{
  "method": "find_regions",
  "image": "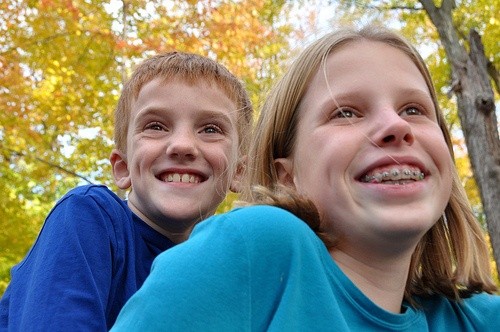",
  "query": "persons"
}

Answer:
[0,51,254,332]
[109,26,500,332]
